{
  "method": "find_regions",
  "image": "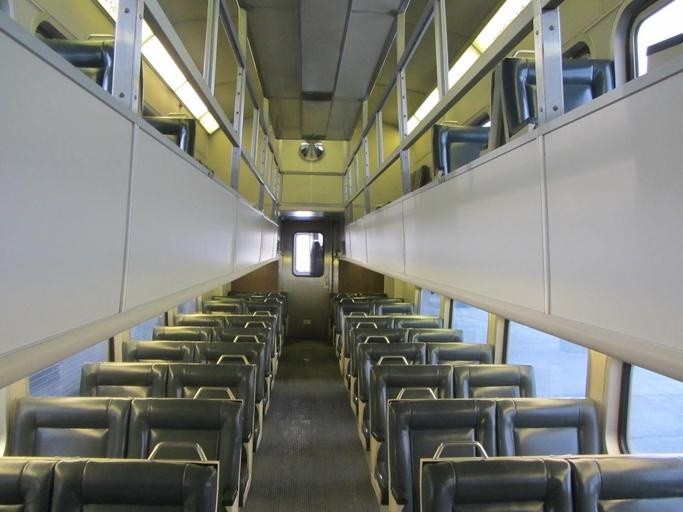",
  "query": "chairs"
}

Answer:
[40,40,114,94]
[437,127,489,175]
[514,56,614,126]
[144,115,194,156]
